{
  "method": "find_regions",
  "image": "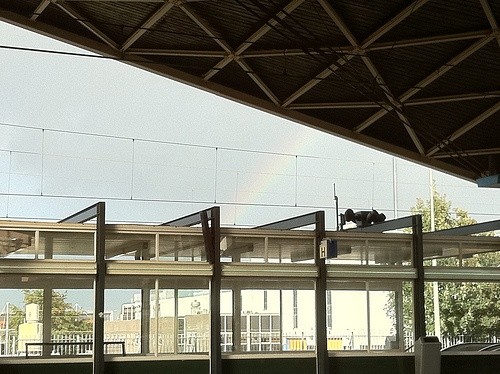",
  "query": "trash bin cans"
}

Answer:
[414,335,442,373]
